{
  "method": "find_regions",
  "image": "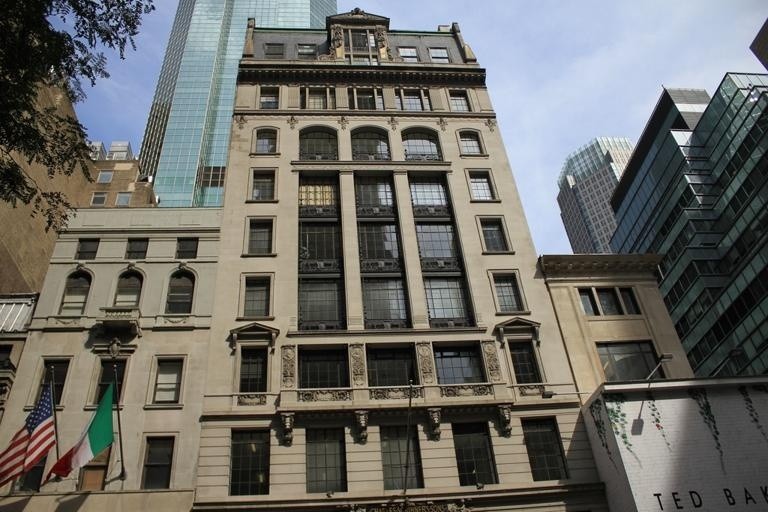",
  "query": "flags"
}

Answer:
[42,380,123,488]
[1,378,60,487]
